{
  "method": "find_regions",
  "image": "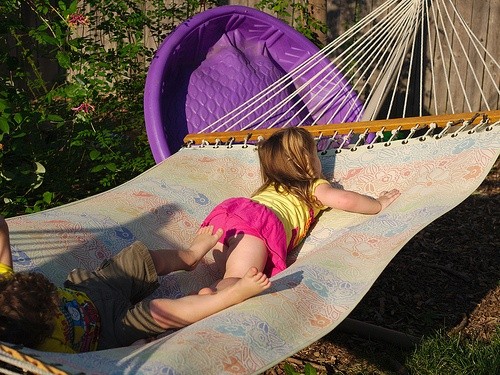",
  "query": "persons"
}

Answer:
[198,127,401,294]
[0,215,270,354]
